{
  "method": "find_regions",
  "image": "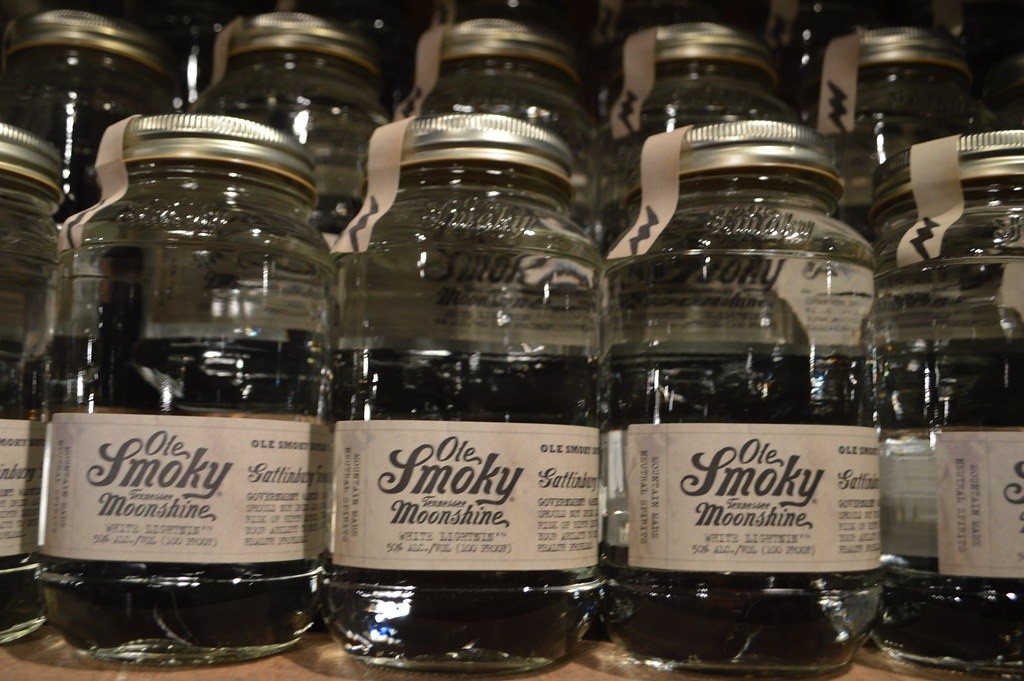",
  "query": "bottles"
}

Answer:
[0,0,1024,681]
